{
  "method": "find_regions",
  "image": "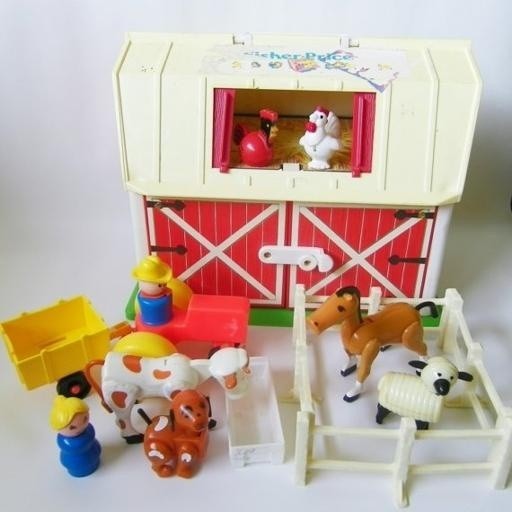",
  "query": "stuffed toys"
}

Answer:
[0,31,512,507]
